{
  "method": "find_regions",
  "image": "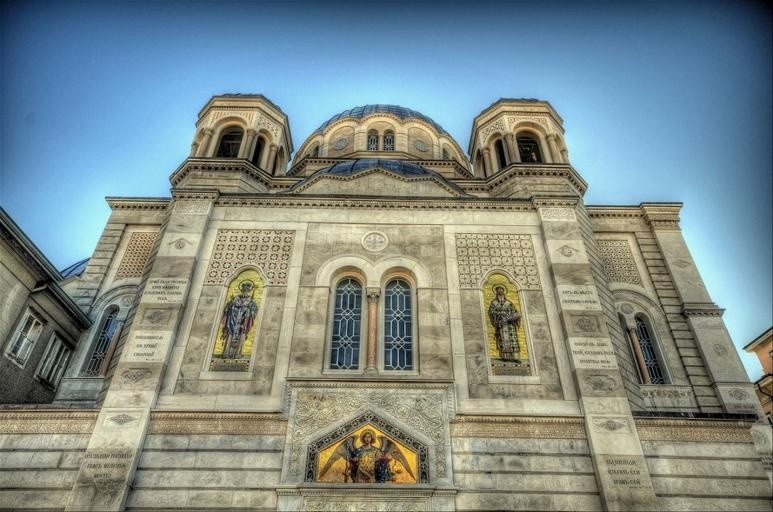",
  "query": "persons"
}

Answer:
[490,283,523,363]
[317,429,415,483]
[217,283,259,359]
